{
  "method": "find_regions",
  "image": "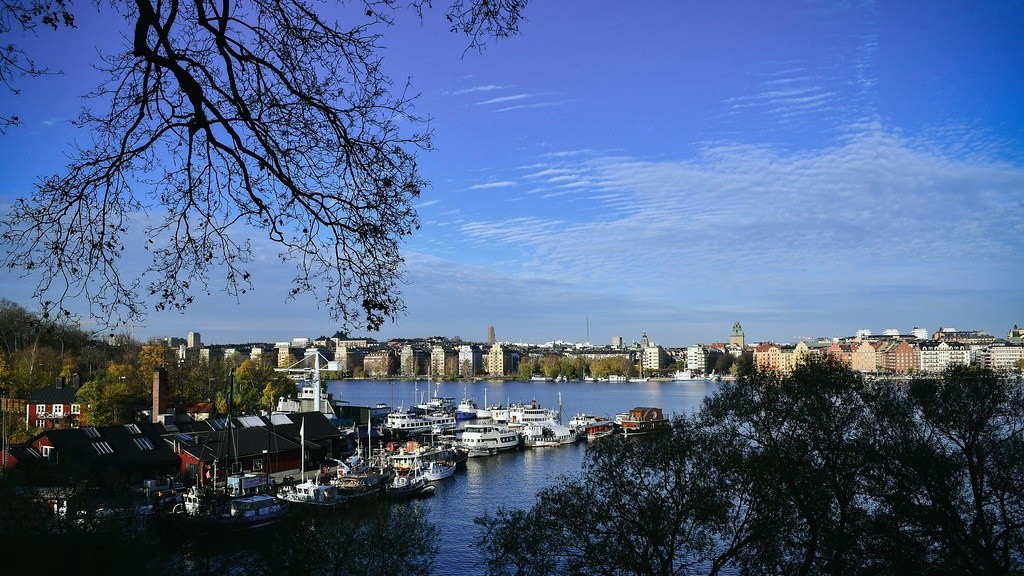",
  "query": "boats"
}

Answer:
[170,395,675,542]
